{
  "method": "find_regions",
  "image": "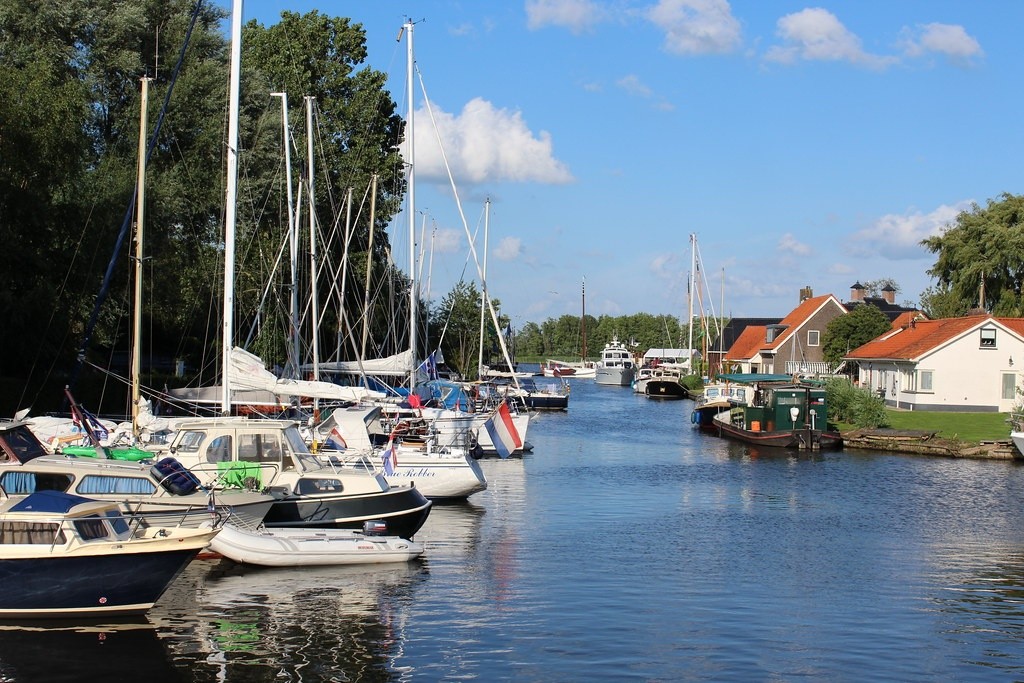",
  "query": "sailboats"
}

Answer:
[1,0,843,620]
